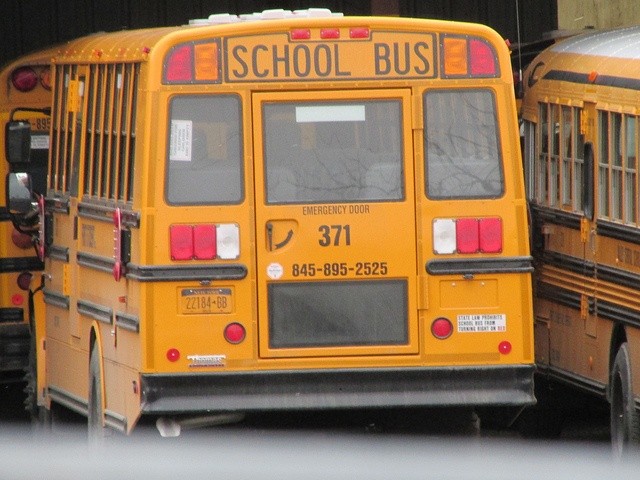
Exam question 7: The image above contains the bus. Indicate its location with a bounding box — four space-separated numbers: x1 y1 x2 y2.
0 46 56 411
26 14 539 471
517 28 640 479
